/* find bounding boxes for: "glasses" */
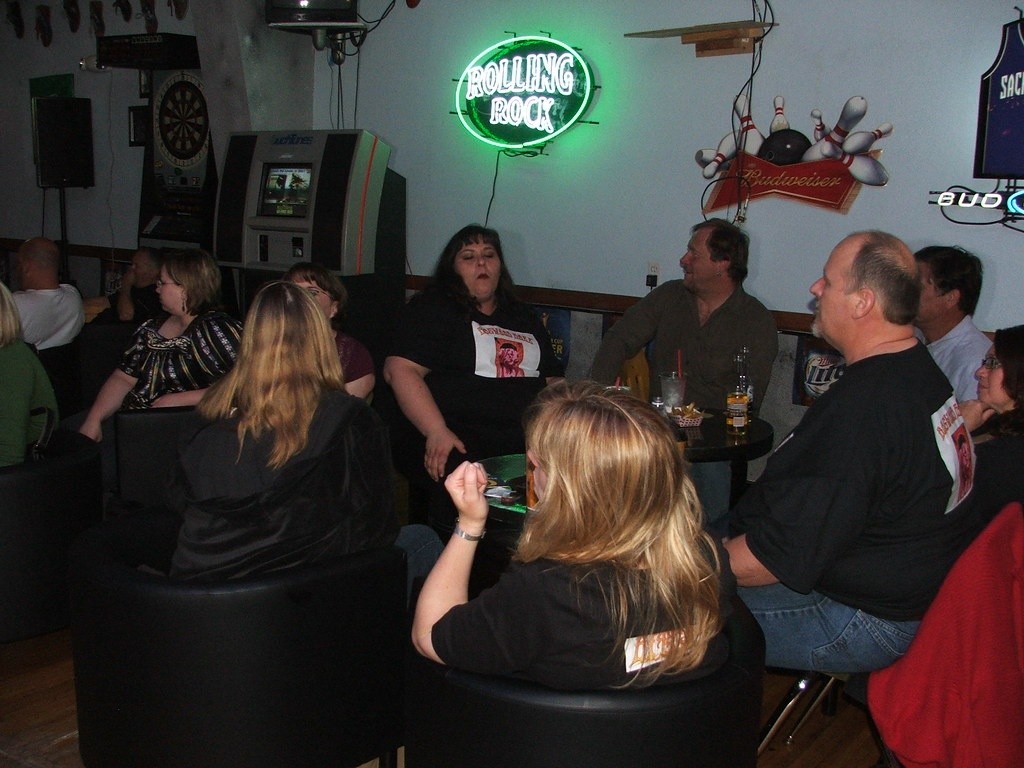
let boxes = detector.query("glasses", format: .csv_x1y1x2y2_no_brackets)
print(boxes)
306,288,333,299
980,357,1001,369
156,279,179,288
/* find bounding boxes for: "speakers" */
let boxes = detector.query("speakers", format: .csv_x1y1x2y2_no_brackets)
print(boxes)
32,96,96,188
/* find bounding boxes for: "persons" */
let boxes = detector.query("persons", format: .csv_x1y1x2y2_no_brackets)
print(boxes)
710,231,982,675
0,235,170,469
79,245,398,580
910,246,1024,505
411,381,736,693
383,223,572,485
585,218,779,522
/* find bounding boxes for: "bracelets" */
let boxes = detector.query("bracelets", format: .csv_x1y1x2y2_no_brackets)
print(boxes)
454,517,487,541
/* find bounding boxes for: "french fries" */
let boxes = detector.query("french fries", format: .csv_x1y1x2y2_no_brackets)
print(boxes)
673,402,705,419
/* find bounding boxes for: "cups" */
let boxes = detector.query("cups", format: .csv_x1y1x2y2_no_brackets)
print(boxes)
605,386,631,392
659,371,686,413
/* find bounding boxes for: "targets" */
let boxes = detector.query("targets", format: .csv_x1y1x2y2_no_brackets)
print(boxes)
158,80,209,158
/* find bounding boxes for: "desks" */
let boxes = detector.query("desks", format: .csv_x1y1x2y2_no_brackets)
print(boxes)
403,434,557,570
675,402,773,515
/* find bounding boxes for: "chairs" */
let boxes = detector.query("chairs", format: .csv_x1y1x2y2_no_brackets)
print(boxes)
1,323,1024,767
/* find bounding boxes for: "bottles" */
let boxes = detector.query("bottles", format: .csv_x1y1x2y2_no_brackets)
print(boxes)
726,355,748,436
652,397,669,421
740,346,753,422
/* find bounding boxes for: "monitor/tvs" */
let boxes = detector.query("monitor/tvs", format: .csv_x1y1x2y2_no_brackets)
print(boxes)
256,162,312,218
263,0,357,23
